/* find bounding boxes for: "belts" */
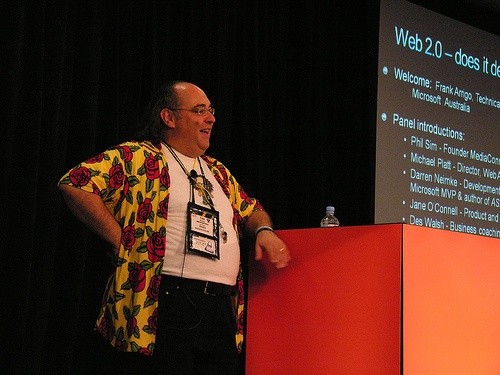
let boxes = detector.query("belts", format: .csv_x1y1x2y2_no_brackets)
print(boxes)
161,275,235,296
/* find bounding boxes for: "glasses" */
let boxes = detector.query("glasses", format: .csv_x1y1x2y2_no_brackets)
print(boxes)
170,106,215,116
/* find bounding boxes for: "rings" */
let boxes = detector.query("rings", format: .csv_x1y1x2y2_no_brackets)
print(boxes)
279,248,284,252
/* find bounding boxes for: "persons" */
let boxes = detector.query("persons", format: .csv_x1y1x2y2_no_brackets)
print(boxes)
57,78,291,375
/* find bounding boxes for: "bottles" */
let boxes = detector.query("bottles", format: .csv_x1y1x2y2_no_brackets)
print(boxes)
320,206,340,228
